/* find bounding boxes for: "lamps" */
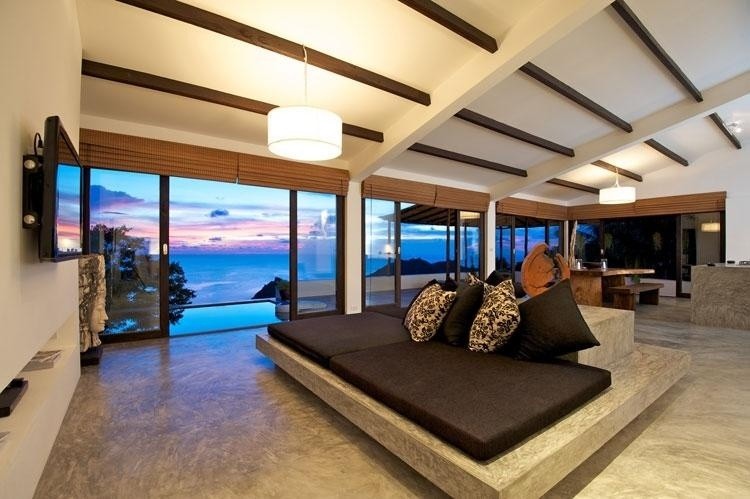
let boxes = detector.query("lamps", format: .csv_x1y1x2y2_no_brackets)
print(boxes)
701,213,720,233
266,45,344,165
599,168,637,205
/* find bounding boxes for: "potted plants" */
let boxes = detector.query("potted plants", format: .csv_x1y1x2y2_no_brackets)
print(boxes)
276,279,299,305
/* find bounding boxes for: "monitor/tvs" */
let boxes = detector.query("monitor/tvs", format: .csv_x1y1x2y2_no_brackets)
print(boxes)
20,114,88,265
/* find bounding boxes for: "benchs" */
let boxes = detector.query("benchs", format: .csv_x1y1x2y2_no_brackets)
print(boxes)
252,307,690,499
607,282,665,312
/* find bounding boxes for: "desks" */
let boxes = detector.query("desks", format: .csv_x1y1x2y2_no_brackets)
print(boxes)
569,268,656,306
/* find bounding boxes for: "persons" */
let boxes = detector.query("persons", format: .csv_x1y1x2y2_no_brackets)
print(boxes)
90,296,110,346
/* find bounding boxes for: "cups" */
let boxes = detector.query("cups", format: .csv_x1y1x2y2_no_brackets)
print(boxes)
576,259,583,270
601,259,608,272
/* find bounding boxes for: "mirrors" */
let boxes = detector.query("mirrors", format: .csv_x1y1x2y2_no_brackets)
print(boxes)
574,223,602,268
680,211,721,294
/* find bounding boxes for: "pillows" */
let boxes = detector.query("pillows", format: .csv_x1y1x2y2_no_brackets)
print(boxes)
405,271,602,362
